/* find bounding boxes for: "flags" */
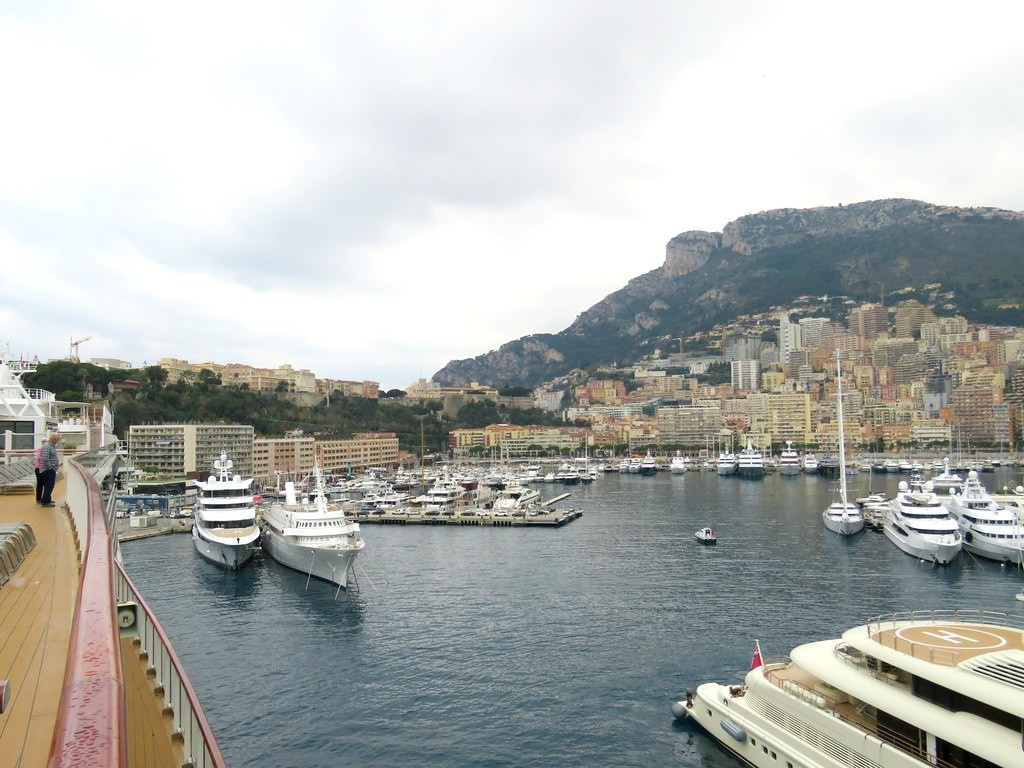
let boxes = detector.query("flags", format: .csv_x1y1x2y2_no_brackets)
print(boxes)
750,641,762,671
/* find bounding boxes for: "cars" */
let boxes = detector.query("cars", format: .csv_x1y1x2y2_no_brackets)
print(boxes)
372,509,386,515
392,508,404,515
494,510,508,516
563,510,570,515
539,506,556,515
116,506,194,518
569,506,575,511
512,510,526,517
406,509,419,516
424,509,440,515
530,510,539,516
441,510,455,515
476,511,490,516
460,511,475,516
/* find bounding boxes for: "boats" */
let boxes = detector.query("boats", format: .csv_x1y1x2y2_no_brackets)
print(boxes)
855,457,1024,565
677,607,1024,768
190,449,260,571
694,528,717,543
259,462,366,590
267,435,819,509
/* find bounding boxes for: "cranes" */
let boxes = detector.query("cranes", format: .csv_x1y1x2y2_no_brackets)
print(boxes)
71,335,92,364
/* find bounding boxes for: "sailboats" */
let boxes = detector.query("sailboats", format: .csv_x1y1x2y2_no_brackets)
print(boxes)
823,348,865,537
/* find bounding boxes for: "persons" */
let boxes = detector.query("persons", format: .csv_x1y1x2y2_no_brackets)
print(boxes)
35,433,61,508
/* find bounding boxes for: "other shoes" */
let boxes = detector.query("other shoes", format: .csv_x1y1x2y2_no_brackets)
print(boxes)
36,499,44,504
41,502,55,507
50,501,56,503
